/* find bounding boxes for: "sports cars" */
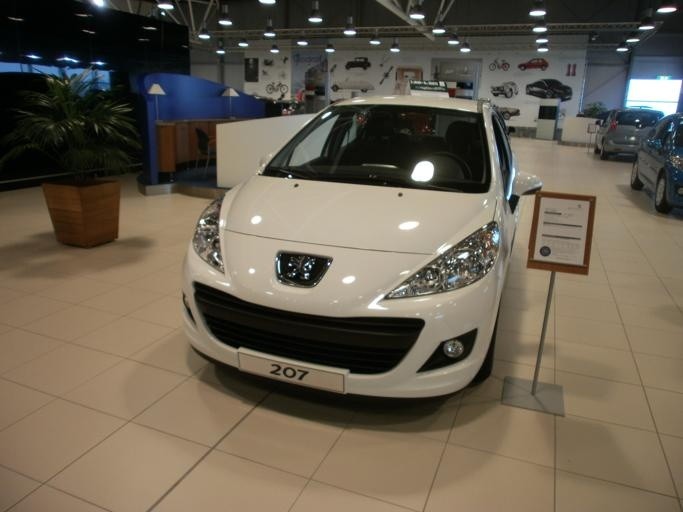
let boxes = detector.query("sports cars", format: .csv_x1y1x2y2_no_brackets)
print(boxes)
330,77,376,93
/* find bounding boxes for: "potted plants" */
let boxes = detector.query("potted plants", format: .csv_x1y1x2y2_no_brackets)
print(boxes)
0,63,144,249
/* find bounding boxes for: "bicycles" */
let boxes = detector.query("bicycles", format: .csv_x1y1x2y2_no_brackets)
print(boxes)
487,52,510,71
266,82,289,94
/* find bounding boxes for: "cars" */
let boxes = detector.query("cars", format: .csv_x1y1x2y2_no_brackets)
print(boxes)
519,58,549,71
492,105,520,119
304,59,325,96
629,112,683,216
526,78,572,102
180,92,542,398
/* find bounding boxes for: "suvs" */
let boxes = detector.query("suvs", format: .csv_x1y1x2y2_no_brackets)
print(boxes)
345,57,371,70
489,80,519,97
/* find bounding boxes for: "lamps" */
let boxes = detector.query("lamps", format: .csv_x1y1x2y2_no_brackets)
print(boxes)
220,88,241,119
147,83,168,122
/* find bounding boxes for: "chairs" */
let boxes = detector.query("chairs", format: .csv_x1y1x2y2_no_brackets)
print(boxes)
192,126,215,176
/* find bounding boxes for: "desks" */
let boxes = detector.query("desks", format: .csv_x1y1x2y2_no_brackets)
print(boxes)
155,117,256,183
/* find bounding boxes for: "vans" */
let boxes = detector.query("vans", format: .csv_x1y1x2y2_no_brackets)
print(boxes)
594,107,663,160
